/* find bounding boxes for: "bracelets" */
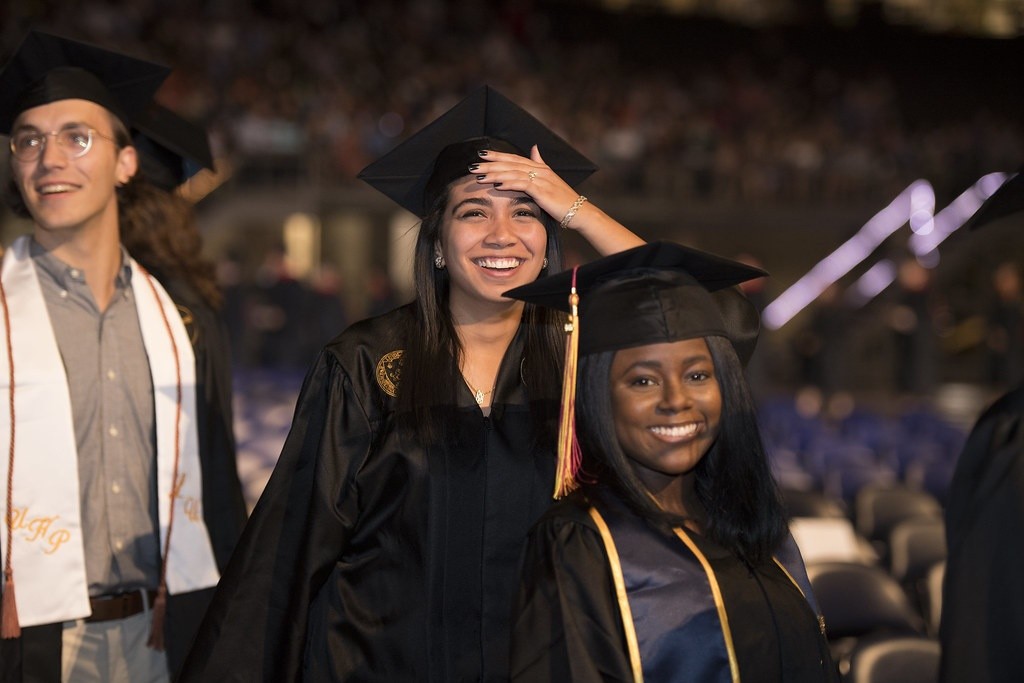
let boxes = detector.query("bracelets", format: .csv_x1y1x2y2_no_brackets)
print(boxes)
559,195,588,230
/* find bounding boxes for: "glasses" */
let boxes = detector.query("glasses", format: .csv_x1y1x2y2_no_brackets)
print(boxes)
9,125,120,162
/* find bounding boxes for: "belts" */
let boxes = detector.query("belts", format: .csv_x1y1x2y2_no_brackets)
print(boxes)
84,590,154,623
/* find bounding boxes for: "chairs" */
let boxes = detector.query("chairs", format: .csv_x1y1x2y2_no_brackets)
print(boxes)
230,367,311,520
753,399,975,683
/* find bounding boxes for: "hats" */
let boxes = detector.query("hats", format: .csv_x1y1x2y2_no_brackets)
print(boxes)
356,85,601,221
131,99,216,192
0,28,172,133
501,239,772,501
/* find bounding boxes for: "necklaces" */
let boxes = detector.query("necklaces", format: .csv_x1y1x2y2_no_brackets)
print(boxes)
461,373,496,402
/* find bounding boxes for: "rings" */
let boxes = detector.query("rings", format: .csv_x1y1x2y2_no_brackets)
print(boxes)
529,171,537,181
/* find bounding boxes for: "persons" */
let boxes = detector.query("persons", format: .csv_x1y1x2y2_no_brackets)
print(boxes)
501,240,837,683
0,30,248,682
185,80,646,683
